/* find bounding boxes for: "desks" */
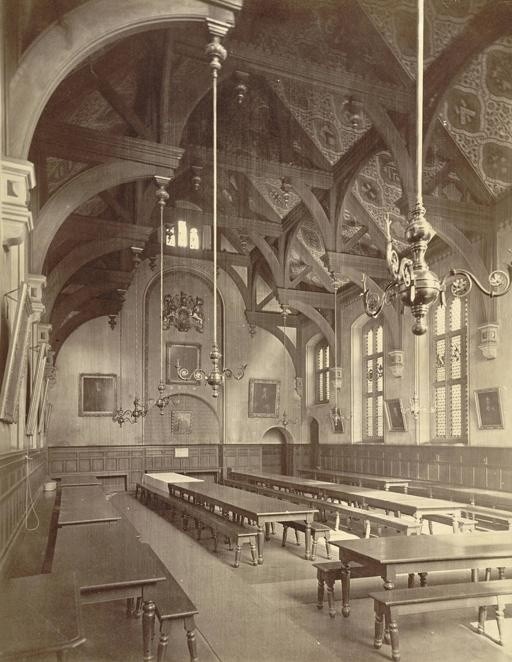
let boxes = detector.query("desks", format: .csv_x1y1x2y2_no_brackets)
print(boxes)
61,475,102,486
425,483,512,510
232,468,470,535
57,485,122,524
141,472,319,560
52,521,167,618
296,467,411,494
327,530,511,618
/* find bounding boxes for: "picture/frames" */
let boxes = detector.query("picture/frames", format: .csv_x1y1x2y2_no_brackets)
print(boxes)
329,408,344,433
384,398,408,432
166,341,201,387
25,343,48,436
78,373,118,417
0,281,34,424
473,387,505,430
248,378,280,419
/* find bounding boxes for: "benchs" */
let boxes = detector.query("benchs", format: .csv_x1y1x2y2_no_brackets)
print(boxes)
400,509,477,534
222,479,422,539
367,579,511,661
135,483,258,567
229,510,331,561
312,561,491,617
126,507,199,662
464,503,512,532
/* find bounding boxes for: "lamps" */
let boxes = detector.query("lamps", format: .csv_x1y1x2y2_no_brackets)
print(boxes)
36,320,53,344
294,376,304,400
387,346,405,378
475,321,500,363
329,366,343,392
25,274,48,304
